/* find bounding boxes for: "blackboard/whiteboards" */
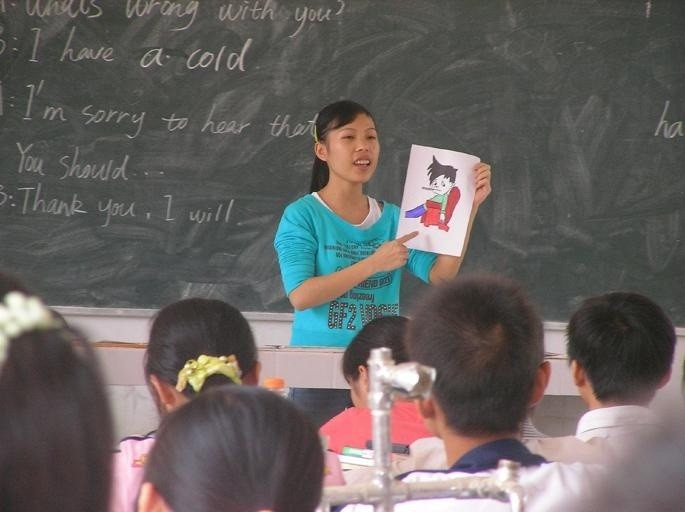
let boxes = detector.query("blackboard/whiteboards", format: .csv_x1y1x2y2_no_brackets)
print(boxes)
0,0,684,338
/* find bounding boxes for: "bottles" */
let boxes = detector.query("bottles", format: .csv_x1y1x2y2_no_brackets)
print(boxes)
262,378,289,398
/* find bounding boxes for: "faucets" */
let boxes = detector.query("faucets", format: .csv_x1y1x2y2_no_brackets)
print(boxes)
367,346,435,476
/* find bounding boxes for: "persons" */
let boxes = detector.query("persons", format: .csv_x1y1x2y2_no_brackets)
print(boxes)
111,296,263,512
524,291,677,462
0,273,117,511
273,98,493,427
318,314,434,455
405,155,458,224
135,383,325,512
333,273,610,511
